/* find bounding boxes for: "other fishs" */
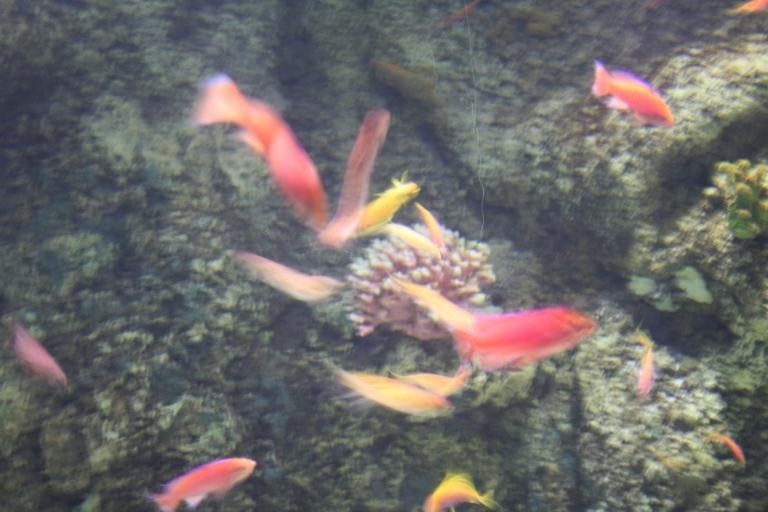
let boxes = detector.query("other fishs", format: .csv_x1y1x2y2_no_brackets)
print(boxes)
193,72,598,421
